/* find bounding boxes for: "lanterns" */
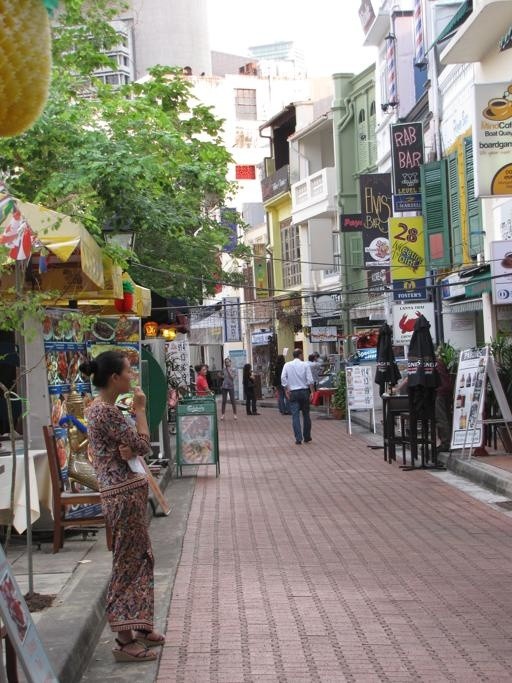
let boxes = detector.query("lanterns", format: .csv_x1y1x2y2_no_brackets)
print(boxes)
159,324,177,341
144,321,158,336
115,280,134,312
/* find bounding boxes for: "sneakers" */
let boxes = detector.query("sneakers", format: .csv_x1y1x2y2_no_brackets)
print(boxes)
295,437,312,444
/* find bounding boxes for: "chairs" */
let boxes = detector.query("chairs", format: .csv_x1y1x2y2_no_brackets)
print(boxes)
42,425,115,553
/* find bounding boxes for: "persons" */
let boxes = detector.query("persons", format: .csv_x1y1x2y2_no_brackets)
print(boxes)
195,365,215,396
220,358,238,420
272,355,292,415
306,352,325,393
243,364,260,415
397,358,454,452
281,348,314,444
79,351,166,662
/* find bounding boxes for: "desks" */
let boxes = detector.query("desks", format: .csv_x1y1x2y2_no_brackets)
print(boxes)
1,448,49,553
382,392,409,463
318,386,337,419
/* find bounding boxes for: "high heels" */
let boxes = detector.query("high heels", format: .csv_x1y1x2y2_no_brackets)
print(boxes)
133,630,167,648
112,637,158,663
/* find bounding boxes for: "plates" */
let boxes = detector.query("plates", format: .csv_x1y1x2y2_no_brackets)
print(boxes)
91,320,115,341
43,325,73,340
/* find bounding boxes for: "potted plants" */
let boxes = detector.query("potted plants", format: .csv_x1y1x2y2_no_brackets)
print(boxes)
330,370,346,420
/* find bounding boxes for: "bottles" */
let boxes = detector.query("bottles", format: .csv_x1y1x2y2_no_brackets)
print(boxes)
456,371,476,430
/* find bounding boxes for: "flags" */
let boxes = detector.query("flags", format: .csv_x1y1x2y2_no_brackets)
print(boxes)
0,180,50,275
